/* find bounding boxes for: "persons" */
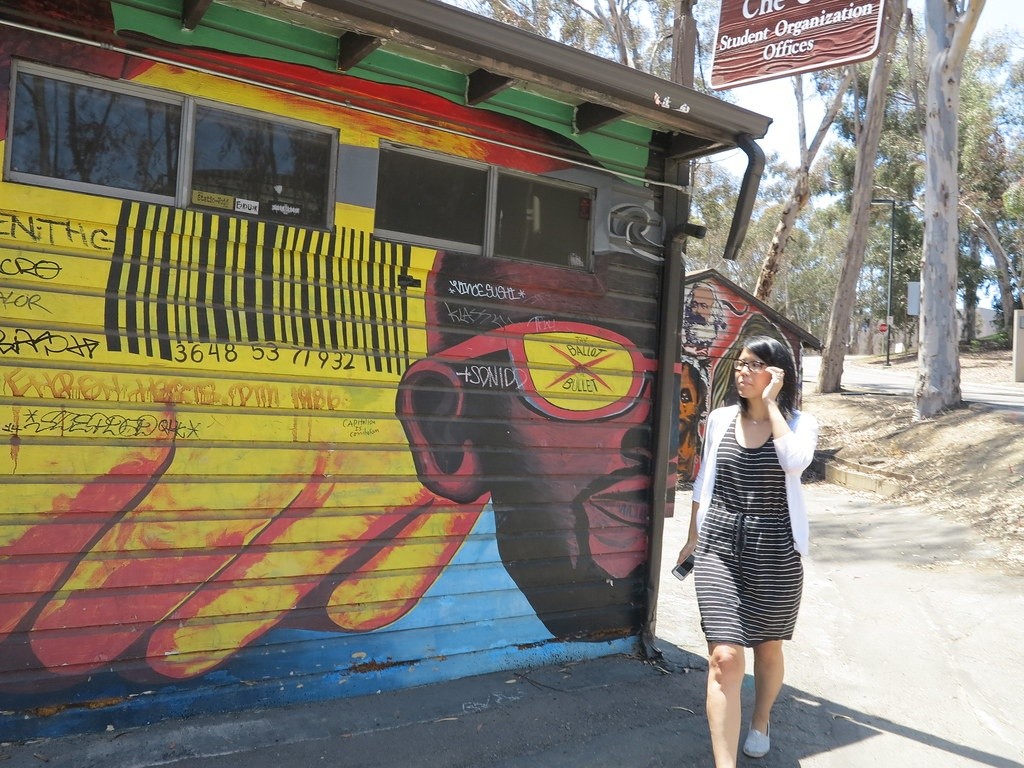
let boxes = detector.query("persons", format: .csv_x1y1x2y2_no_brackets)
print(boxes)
676,334,818,768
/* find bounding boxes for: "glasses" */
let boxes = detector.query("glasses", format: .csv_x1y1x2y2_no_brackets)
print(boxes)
733,358,768,372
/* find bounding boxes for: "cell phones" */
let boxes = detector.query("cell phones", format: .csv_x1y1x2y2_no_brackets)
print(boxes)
672,551,696,580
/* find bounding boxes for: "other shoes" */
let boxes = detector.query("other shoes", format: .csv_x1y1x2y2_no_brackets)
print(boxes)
743,716,771,758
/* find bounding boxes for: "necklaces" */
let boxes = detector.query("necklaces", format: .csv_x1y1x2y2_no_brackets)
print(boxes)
745,413,769,425
